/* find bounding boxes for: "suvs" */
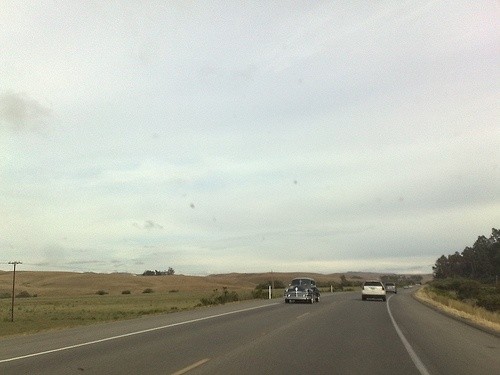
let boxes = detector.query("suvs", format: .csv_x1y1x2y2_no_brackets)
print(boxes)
362,280,386,300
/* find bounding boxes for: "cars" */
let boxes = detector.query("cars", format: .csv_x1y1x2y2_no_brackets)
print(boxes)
384,282,396,294
284,277,320,304
